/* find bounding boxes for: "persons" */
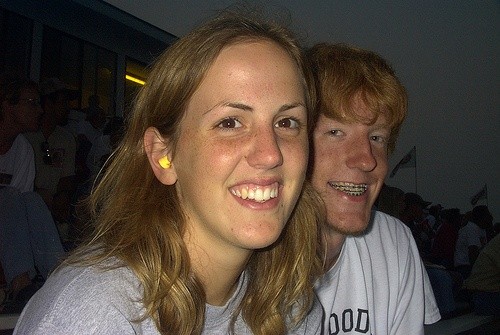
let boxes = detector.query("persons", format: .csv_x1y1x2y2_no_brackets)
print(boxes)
0,78,500,335
307,42,442,335
14,12,325,335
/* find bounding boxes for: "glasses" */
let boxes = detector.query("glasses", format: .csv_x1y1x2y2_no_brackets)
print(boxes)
12,98,42,106
41,141,52,165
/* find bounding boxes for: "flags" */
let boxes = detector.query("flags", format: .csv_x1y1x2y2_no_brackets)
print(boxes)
471,185,487,206
390,147,416,179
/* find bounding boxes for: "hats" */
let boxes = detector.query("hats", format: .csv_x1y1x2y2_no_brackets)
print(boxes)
405,193,432,207
